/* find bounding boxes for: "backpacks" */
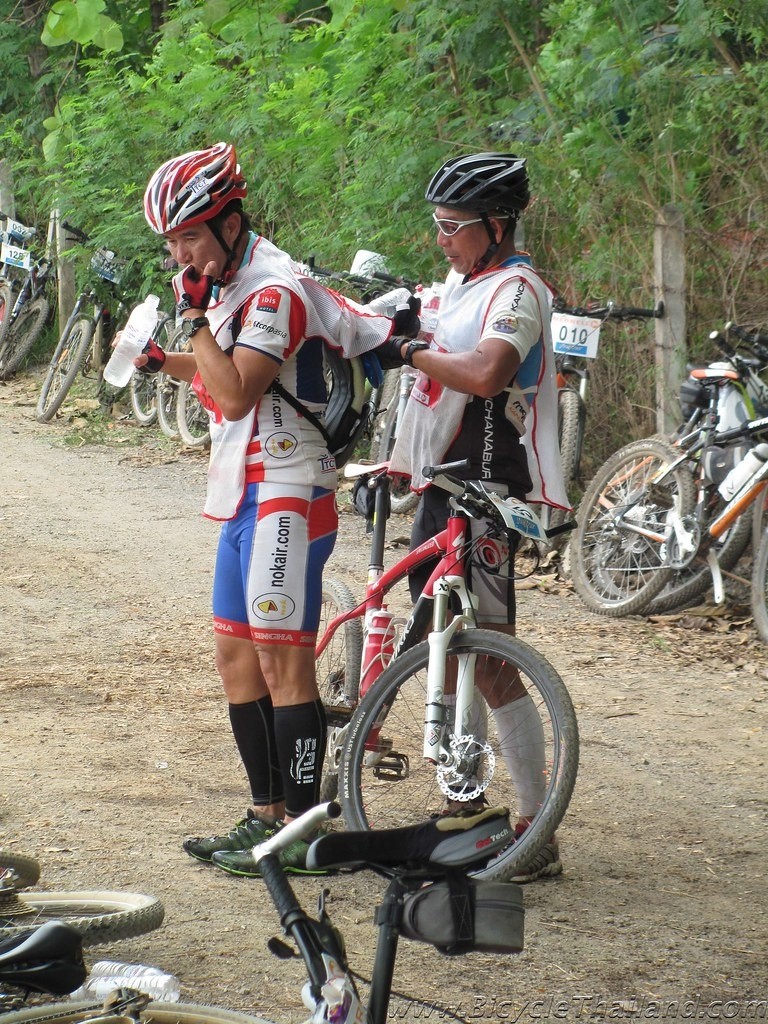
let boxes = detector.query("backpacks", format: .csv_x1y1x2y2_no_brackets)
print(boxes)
230,277,385,469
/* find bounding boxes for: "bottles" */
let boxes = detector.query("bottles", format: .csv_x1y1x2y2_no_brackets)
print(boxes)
718,442,768,501
70,961,181,1005
102,294,160,387
368,288,413,318
359,604,394,699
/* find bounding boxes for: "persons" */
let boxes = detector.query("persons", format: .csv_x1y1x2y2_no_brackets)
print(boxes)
112,141,340,877
377,151,572,882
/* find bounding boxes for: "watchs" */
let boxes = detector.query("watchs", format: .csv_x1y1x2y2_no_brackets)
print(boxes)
406,339,430,369
182,316,209,337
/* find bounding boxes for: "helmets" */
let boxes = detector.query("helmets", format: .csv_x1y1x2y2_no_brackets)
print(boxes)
142,140,249,260
425,152,531,285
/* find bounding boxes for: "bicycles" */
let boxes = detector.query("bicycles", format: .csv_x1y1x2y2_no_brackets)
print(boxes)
1,798,532,1023
307,455,579,884
0,216,767,640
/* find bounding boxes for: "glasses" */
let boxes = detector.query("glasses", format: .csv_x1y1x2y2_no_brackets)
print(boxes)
429,211,509,236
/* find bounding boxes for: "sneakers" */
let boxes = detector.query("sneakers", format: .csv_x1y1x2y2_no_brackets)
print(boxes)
430,809,451,820
498,824,563,882
183,808,340,878
